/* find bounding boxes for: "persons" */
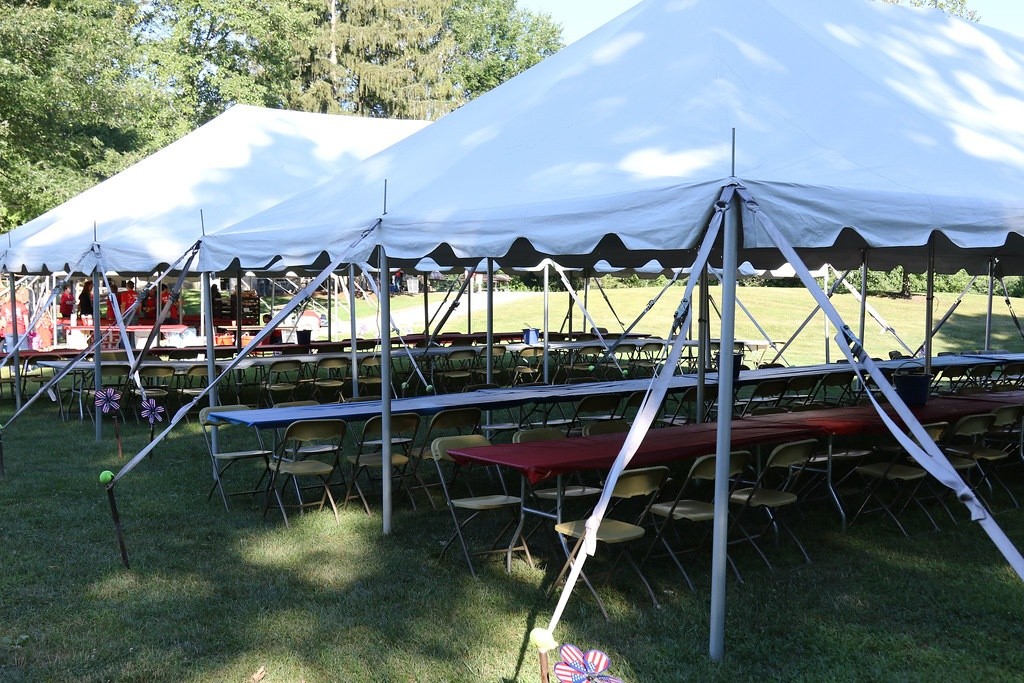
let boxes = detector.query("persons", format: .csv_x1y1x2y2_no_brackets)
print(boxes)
161,283,179,323
1,287,35,375
79,281,94,364
106,285,119,348
60,284,77,340
120,280,141,362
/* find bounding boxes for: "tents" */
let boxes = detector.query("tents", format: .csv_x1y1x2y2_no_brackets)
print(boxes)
197,0,1022,659
0,102,761,481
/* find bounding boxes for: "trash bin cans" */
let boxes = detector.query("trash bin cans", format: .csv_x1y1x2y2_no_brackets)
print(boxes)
6,333,29,372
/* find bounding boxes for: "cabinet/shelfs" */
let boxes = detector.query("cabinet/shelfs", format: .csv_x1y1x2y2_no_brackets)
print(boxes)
231,294,260,326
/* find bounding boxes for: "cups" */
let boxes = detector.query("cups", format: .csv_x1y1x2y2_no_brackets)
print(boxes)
232,320,236,326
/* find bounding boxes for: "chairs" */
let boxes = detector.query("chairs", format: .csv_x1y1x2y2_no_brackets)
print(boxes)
0,328,1024,624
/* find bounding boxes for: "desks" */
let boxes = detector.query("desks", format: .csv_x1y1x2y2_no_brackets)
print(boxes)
0,344,243,400
57,315,299,350
207,391,557,515
872,355,1007,390
446,420,815,570
35,358,254,422
391,341,549,371
947,390,1024,461
540,333,651,340
676,363,864,390
550,339,744,354
243,353,381,366
477,376,718,430
256,335,442,357
962,352,1024,385
442,332,540,341
745,398,1019,535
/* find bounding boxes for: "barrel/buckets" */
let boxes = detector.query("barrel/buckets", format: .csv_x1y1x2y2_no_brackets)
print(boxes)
522,328,541,346
216,333,257,347
406,278,420,294
296,330,312,344
